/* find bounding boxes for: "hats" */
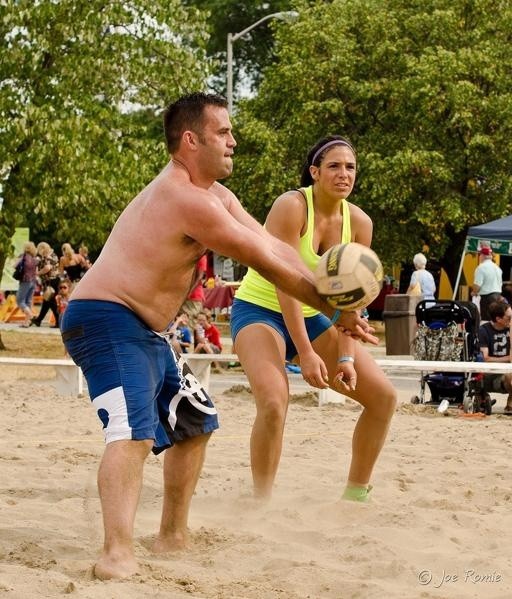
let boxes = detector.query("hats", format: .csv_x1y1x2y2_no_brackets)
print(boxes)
476,247,493,256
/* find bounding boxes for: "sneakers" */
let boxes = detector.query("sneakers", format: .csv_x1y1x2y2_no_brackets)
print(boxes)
19,315,60,329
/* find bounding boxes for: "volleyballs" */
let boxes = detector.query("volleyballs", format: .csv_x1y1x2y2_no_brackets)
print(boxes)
314,242,385,312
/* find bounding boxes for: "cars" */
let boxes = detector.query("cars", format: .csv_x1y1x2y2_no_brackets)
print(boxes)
367,271,400,320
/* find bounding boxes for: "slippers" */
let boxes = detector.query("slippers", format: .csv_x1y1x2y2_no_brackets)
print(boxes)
491,398,497,405
504,405,512,412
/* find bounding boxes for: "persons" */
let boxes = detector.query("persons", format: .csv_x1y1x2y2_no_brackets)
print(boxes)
472,247,512,411
167,254,223,370
15,242,93,331
227,132,399,507
58,92,382,581
406,253,436,309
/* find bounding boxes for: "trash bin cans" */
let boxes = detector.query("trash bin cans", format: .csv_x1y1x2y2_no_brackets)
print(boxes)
382,294,424,355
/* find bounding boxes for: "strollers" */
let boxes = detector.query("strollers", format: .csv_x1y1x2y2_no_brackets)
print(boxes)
407,297,498,417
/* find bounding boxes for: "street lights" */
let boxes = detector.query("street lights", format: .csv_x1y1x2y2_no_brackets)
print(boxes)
224,10,300,114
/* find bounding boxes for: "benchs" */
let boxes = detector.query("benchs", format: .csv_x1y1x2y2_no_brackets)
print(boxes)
174,353,510,422
1,354,87,399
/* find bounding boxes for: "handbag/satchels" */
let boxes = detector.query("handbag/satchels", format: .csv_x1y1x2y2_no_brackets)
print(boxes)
42,284,57,303
406,279,423,315
411,322,468,364
12,253,27,281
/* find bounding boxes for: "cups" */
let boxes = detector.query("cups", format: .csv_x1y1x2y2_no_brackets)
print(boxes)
480,348,489,358
